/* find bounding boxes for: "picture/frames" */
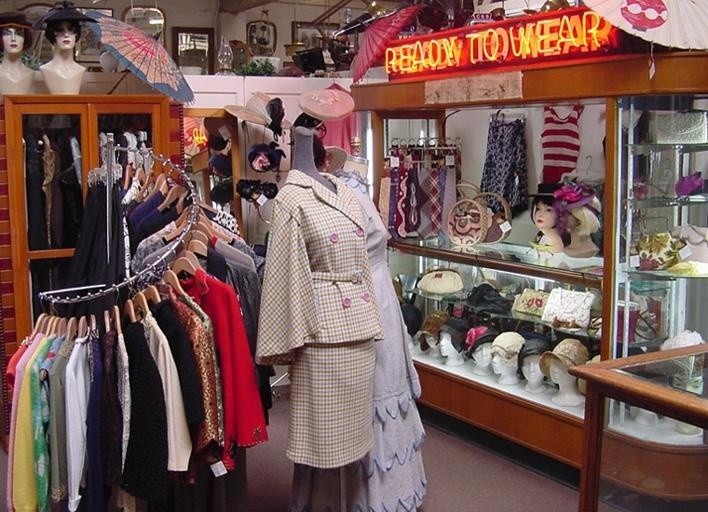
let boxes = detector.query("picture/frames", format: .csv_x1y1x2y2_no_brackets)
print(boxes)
292,21,340,48
69,8,114,65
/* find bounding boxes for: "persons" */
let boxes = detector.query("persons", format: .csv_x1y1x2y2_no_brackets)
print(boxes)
39,10,98,95
1,11,37,95
398,305,589,409
553,183,601,259
254,126,381,512
528,181,565,252
323,147,430,512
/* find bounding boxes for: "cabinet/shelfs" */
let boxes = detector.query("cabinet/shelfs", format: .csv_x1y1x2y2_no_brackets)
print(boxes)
2,91,171,344
568,342,706,509
350,49,707,500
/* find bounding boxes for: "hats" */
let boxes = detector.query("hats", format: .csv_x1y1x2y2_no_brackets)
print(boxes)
0,12,34,29
538,338,589,378
313,139,347,173
518,336,552,380
463,323,500,357
529,181,565,203
398,301,470,353
44,8,100,24
578,355,602,396
224,89,292,132
490,331,525,359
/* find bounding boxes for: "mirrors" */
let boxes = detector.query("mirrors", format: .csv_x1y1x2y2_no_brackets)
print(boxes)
171,26,215,76
181,107,246,240
120,6,166,49
246,21,277,58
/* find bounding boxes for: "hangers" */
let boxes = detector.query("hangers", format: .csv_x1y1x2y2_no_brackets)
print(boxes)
6,258,215,384
114,143,256,277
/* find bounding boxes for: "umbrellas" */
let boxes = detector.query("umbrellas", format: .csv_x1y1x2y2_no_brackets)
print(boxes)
351,5,424,82
84,10,196,104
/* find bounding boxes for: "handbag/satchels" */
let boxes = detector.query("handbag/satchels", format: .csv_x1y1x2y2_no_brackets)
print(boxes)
668,221,707,265
466,280,513,313
636,218,680,271
615,299,656,346
539,286,595,337
648,108,708,146
509,286,550,323
